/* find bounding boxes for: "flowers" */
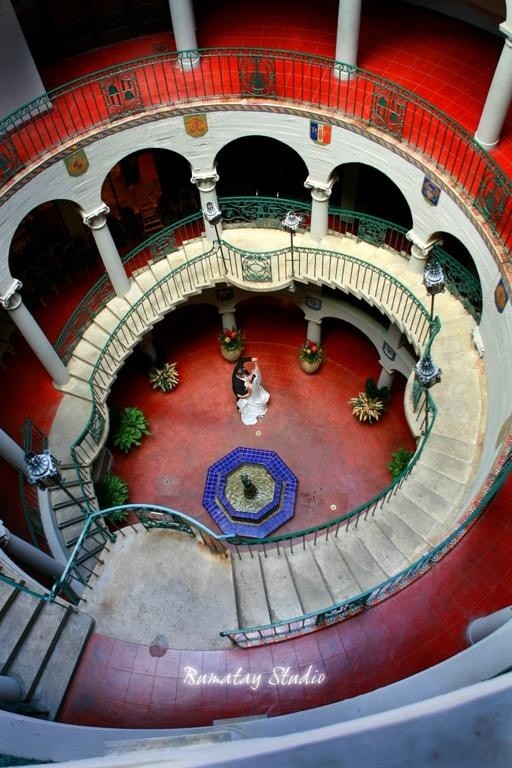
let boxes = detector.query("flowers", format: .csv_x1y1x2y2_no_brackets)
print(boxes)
217,328,244,352
298,338,322,363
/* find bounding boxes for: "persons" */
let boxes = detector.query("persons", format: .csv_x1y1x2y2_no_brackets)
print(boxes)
232,355,258,407
234,362,271,426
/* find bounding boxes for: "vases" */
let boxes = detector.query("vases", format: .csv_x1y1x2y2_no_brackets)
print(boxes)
300,358,320,374
220,344,241,362
